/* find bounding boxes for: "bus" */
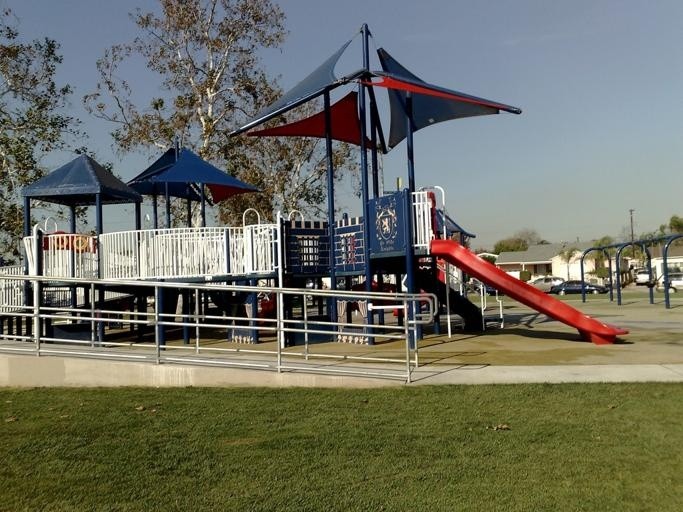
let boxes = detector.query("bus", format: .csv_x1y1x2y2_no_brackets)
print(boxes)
655,257,682,293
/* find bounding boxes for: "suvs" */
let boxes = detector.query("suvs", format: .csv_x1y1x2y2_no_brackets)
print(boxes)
527,276,564,294
635,270,653,285
655,272,682,294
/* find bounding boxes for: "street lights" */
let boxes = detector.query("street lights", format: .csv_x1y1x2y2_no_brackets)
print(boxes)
629,208,634,260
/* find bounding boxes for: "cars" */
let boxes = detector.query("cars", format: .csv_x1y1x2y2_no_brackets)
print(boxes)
484,284,500,294
550,280,608,295
